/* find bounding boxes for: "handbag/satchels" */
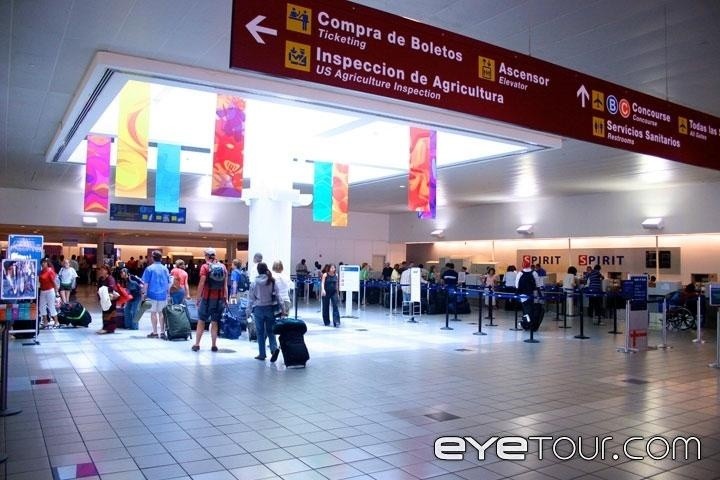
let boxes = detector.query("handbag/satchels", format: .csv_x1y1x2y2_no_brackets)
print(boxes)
108,290,120,301
170,275,180,293
271,317,307,335
57,313,69,325
271,279,290,323
217,302,241,340
115,282,134,305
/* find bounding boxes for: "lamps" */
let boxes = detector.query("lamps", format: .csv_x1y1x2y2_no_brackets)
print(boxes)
430,229,445,239
82,216,98,226
641,218,664,230
199,222,214,231
516,225,533,235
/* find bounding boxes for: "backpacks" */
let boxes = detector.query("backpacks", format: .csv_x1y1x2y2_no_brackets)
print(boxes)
518,270,536,295
237,270,251,292
205,261,226,290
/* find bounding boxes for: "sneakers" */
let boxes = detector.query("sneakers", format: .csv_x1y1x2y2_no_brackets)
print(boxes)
192,345,200,352
255,355,265,360
160,332,166,338
270,348,279,362
146,332,159,338
212,346,218,351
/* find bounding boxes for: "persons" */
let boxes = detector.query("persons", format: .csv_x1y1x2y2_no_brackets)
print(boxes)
39,246,655,362
666,283,697,320
3,261,20,297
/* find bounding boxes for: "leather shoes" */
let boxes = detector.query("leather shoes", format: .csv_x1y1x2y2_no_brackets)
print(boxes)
96,329,114,333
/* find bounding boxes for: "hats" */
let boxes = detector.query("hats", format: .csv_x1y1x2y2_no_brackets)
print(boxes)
203,247,217,258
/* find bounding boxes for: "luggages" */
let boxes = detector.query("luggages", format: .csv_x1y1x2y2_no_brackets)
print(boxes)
225,302,248,331
162,298,193,340
115,307,125,328
279,313,310,368
247,319,267,342
55,291,92,328
520,303,545,331
